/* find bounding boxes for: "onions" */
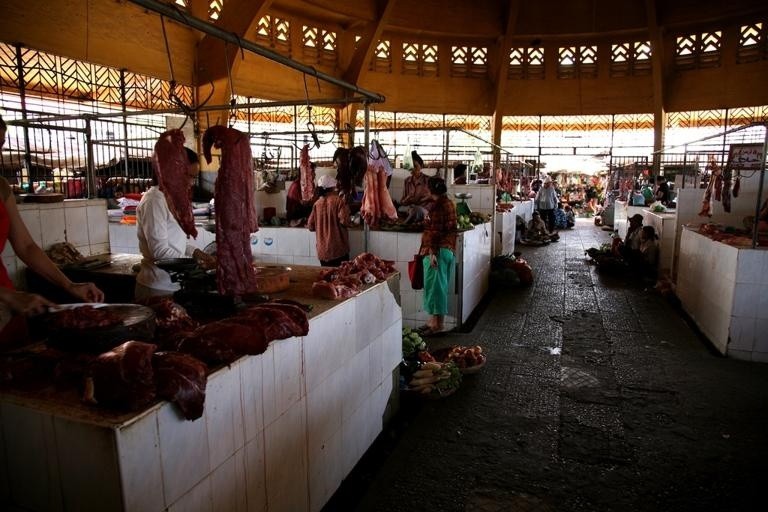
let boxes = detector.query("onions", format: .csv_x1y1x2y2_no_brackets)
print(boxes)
445,344,483,365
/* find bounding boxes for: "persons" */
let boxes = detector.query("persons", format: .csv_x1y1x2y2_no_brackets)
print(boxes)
622,214,643,251
654,176,670,203
534,176,560,234
408,175,457,337
134,147,216,306
626,225,660,297
286,163,325,219
527,211,560,242
0,116,105,332
553,202,568,230
307,175,356,267
400,150,437,212
564,205,575,228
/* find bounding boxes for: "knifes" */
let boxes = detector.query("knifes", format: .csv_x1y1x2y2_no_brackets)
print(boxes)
43,305,73,314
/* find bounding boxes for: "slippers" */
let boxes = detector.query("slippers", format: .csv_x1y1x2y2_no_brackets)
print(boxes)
418,323,448,338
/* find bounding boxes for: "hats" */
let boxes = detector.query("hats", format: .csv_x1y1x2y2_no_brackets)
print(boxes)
317,175,338,189
627,213,644,227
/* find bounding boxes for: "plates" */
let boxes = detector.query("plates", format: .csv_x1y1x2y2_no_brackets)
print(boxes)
18,192,66,203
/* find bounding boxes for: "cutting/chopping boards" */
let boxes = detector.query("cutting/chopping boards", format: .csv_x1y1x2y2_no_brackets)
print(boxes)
57,303,157,342
254,267,290,294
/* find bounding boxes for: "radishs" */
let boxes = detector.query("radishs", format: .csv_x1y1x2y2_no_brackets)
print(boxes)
409,361,452,394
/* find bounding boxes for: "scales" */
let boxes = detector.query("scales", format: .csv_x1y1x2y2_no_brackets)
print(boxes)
151,257,234,319
455,193,472,216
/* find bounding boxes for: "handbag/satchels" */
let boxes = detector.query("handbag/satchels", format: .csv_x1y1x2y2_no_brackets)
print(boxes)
407,242,424,290
